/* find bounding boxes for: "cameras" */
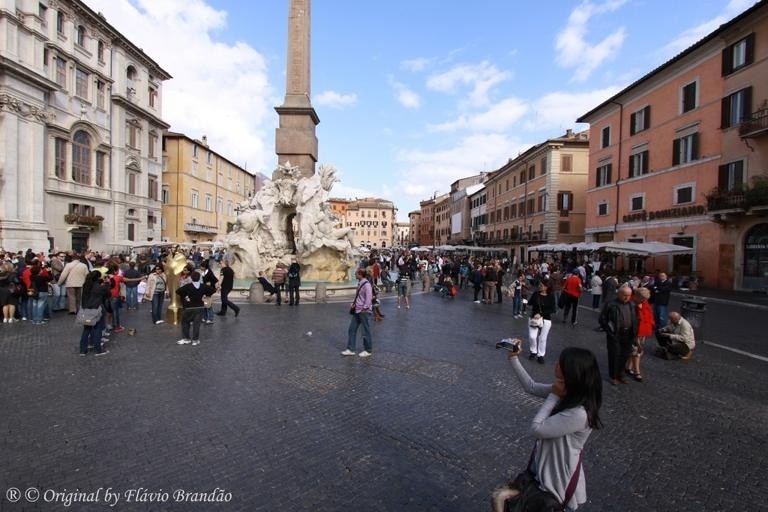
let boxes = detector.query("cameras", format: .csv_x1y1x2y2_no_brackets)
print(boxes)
496,338,519,353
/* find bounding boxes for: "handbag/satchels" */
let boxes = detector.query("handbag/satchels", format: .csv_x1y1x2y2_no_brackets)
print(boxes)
74,306,103,326
491,469,565,512
349,304,356,315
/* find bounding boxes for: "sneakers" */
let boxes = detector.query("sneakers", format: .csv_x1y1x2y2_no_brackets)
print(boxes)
610,375,632,385
472,298,502,305
528,353,545,364
93,350,110,357
2,316,49,327
405,305,409,309
79,349,90,357
340,348,356,357
396,305,401,309
87,324,125,349
624,367,644,382
176,338,192,345
216,311,226,316
192,339,200,346
201,317,215,324
512,310,528,321
234,307,240,317
155,319,165,325
51,308,78,315
358,350,372,357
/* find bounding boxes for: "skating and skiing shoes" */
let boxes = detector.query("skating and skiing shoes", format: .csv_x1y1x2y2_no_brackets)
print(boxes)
373,313,385,321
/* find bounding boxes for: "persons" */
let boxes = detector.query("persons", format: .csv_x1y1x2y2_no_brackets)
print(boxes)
0,245,240,356
289,258,301,305
283,264,291,303
598,285,640,385
341,268,373,357
492,337,602,511
657,311,696,360
271,263,285,306
257,271,276,303
527,279,556,362
228,165,356,280
625,286,656,382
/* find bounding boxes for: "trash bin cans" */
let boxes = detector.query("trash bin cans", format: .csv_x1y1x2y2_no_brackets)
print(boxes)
680,299,708,344
